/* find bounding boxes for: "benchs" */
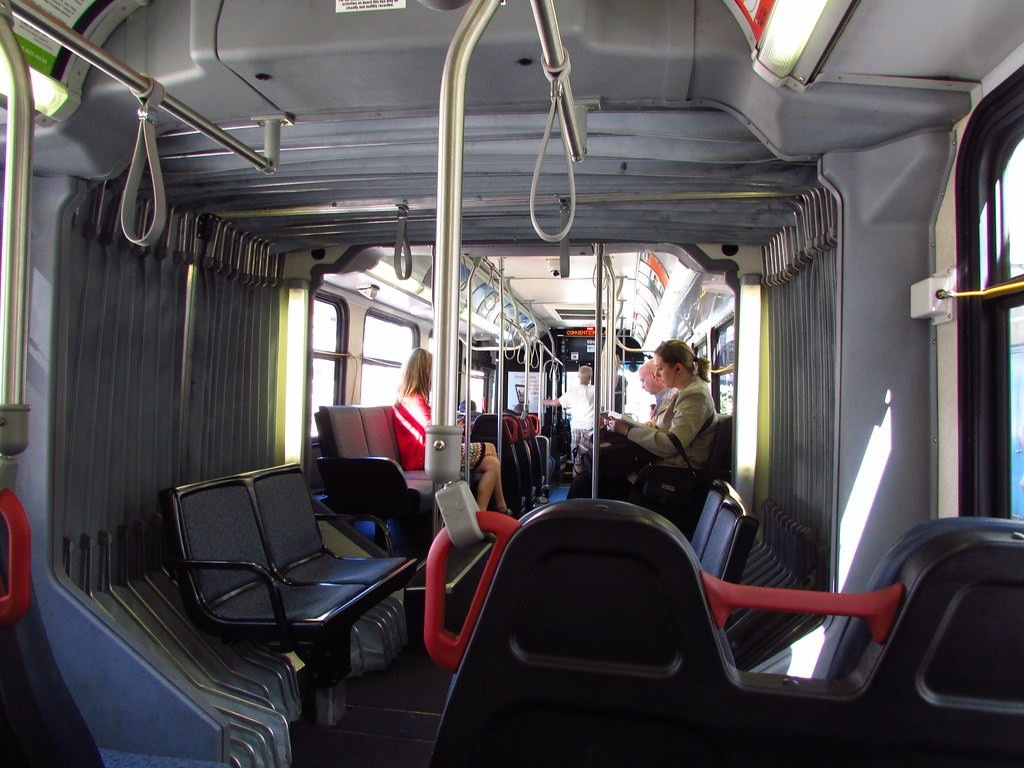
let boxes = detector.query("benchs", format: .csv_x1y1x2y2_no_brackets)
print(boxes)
162,412,1024,768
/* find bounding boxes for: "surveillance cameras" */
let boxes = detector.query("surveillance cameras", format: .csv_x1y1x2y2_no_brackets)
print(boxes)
551,270,559,277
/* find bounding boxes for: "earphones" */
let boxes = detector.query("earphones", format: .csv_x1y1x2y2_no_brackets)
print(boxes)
676,367,680,371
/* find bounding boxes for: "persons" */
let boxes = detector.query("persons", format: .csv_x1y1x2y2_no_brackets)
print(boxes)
543,366,595,477
566,340,718,498
459,400,476,412
514,403,524,413
615,351,627,414
391,348,513,517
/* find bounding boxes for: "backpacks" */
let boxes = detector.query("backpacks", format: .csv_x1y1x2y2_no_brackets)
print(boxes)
572,425,654,480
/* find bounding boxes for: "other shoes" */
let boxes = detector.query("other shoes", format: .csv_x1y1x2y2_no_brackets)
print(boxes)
538,496,549,504
484,532,496,544
494,506,513,517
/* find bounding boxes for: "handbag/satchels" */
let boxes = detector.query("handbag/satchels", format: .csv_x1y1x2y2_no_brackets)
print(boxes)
644,433,701,497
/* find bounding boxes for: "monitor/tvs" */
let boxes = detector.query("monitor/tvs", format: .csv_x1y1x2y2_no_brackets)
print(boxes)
515,384,530,403
